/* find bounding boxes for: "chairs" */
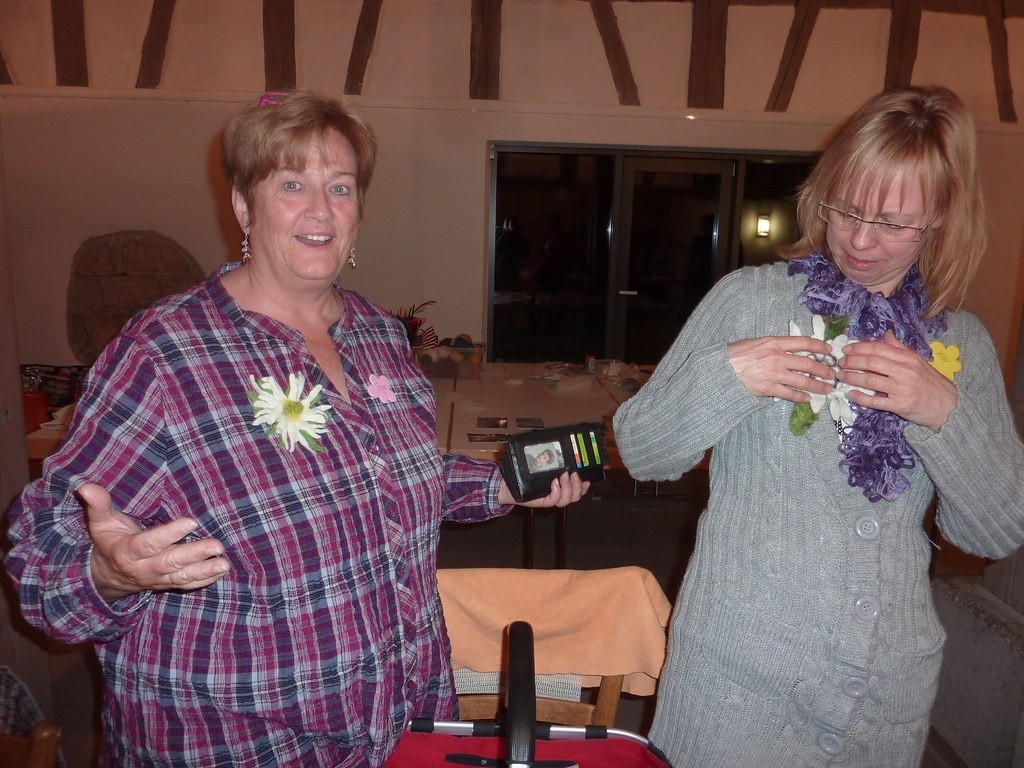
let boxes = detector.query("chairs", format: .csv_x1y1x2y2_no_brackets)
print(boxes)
433,563,648,730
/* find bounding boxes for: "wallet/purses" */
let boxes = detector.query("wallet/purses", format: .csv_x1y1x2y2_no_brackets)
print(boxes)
497,423,607,503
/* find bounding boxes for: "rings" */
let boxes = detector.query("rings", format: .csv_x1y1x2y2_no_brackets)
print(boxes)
557,503,561,508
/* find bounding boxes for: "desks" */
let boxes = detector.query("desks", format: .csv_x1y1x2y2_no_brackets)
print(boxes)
429,363,713,570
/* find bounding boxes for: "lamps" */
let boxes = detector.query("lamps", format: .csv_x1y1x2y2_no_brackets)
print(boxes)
757,213,772,237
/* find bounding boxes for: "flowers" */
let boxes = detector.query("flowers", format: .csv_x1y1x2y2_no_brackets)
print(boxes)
243,372,334,454
783,316,876,433
927,342,963,385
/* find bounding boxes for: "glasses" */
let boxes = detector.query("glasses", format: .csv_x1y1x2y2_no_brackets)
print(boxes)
818,190,942,243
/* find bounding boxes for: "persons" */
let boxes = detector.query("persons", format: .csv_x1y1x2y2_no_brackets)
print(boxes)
0,91,590,768
613,87,1024,768
525,450,556,473
